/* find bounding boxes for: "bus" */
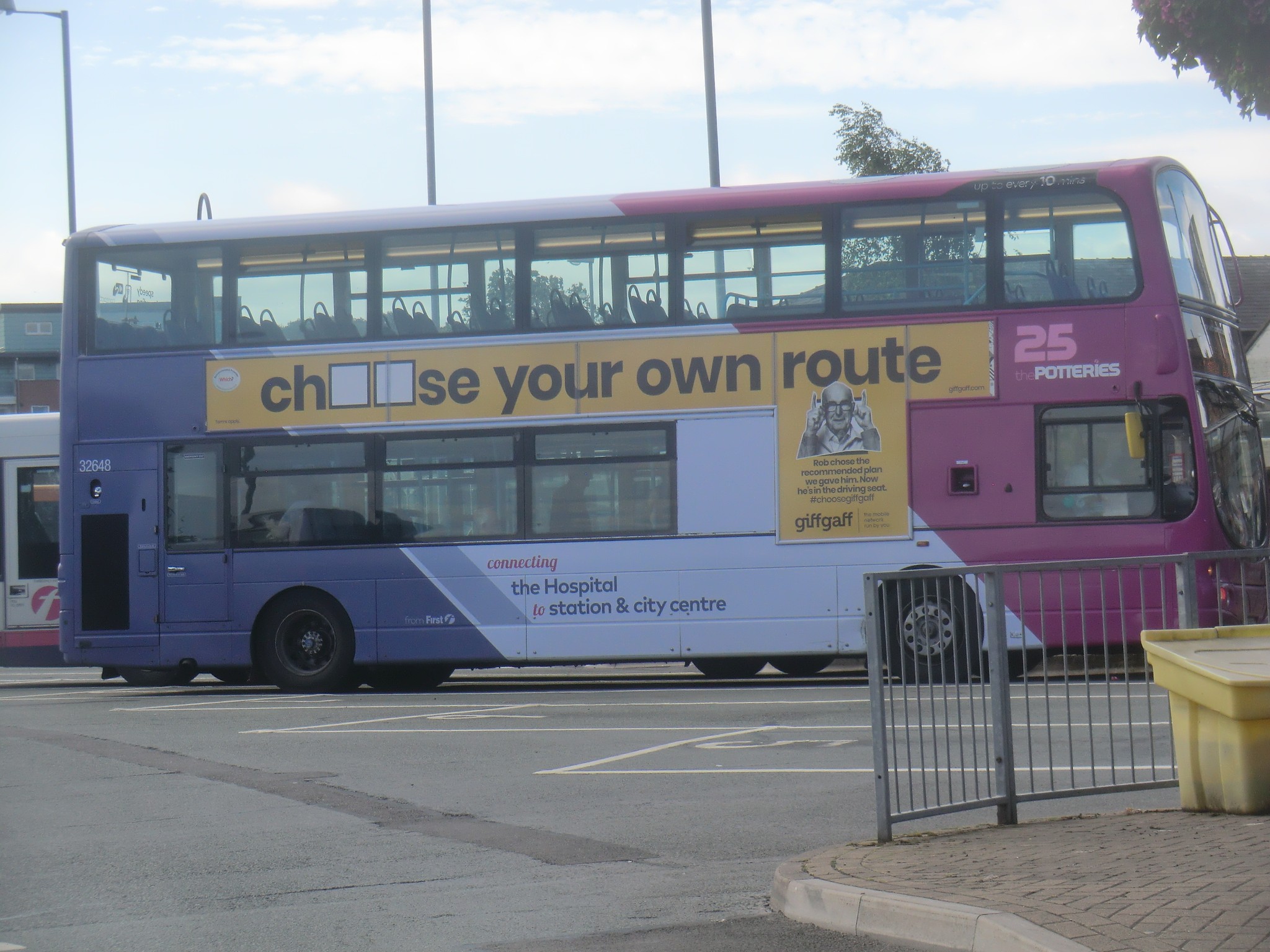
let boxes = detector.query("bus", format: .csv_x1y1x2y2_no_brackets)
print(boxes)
46,152,1270,702
0,411,64,666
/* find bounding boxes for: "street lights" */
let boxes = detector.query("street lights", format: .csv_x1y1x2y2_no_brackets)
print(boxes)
1,0,76,238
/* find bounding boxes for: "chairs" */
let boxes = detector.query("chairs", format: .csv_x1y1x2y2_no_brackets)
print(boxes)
93,279,1107,352
241,497,665,542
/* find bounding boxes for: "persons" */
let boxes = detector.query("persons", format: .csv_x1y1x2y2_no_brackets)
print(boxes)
797,381,881,459
1063,437,1136,519
